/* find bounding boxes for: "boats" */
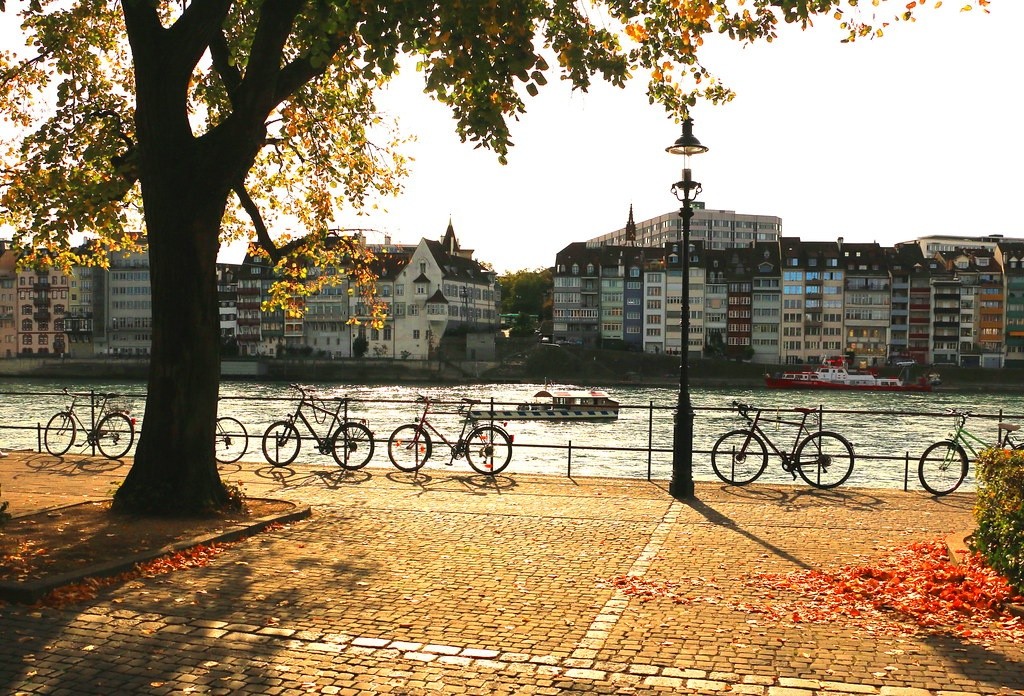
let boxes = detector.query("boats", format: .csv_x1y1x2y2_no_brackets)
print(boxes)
764,355,942,393
461,376,620,421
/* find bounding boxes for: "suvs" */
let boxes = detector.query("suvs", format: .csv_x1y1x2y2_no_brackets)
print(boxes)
555,336,583,347
895,359,916,367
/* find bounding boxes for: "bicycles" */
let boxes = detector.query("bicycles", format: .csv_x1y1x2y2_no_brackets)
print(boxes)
711,400,855,490
214,397,248,465
44,387,137,461
388,393,515,476
261,383,375,471
917,408,1024,496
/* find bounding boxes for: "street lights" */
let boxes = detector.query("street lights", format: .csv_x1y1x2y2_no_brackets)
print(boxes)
663,112,709,498
